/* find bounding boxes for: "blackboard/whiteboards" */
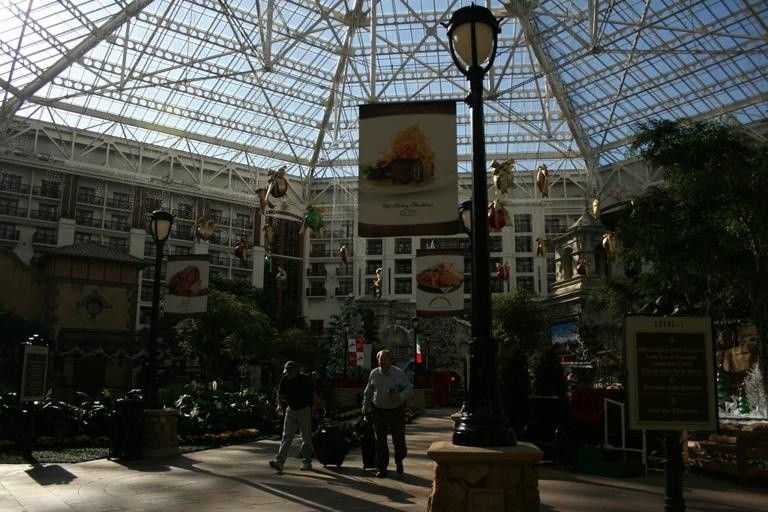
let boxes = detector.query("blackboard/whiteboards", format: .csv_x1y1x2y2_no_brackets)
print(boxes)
603,397,626,450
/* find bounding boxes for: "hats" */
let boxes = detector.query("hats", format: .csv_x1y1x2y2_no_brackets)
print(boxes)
283,361,297,373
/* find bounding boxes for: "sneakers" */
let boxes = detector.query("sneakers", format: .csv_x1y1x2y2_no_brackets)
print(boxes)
269,459,283,472
299,463,312,470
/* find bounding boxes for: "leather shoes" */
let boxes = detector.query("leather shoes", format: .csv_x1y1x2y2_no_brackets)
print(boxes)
397,465,403,474
376,471,388,478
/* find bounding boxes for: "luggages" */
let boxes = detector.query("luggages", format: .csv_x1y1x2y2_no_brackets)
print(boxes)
311,414,347,467
362,441,378,471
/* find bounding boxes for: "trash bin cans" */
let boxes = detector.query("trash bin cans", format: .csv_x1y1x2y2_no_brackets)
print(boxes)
110,399,145,462
453,412,492,443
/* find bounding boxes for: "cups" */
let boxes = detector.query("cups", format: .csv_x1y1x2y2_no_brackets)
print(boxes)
386,387,396,401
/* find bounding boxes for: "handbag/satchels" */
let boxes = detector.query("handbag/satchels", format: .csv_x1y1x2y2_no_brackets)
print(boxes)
352,413,375,443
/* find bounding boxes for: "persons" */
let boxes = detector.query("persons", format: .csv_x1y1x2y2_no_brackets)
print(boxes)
362,349,413,477
270,361,313,471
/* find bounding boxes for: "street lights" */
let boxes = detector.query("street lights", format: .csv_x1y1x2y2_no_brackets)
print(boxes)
337,319,351,387
138,204,178,409
458,185,478,247
433,0,528,446
